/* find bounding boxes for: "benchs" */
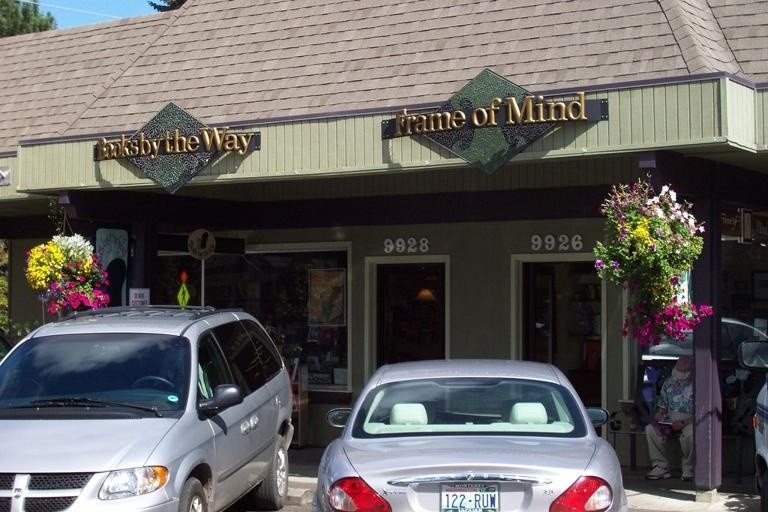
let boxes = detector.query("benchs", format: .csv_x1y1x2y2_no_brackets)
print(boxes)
609,405,756,485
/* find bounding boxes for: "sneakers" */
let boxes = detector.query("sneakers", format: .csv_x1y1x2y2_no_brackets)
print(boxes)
646,465,671,480
680,472,694,480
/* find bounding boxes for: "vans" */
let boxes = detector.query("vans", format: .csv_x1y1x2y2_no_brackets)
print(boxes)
0,300,295,511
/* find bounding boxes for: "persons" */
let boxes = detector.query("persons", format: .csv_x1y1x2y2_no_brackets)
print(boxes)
645,354,696,480
148,338,192,393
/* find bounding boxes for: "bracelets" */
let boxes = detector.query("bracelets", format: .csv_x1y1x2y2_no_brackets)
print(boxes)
680,420,689,426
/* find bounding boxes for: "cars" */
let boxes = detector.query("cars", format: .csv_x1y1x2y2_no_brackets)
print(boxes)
316,358,629,512
647,309,767,394
738,336,768,512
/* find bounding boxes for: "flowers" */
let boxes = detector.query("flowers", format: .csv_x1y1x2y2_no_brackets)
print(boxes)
593,169,716,350
21,230,113,319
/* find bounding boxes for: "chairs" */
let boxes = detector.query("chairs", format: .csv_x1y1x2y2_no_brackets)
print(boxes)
509,400,549,426
389,402,428,425
